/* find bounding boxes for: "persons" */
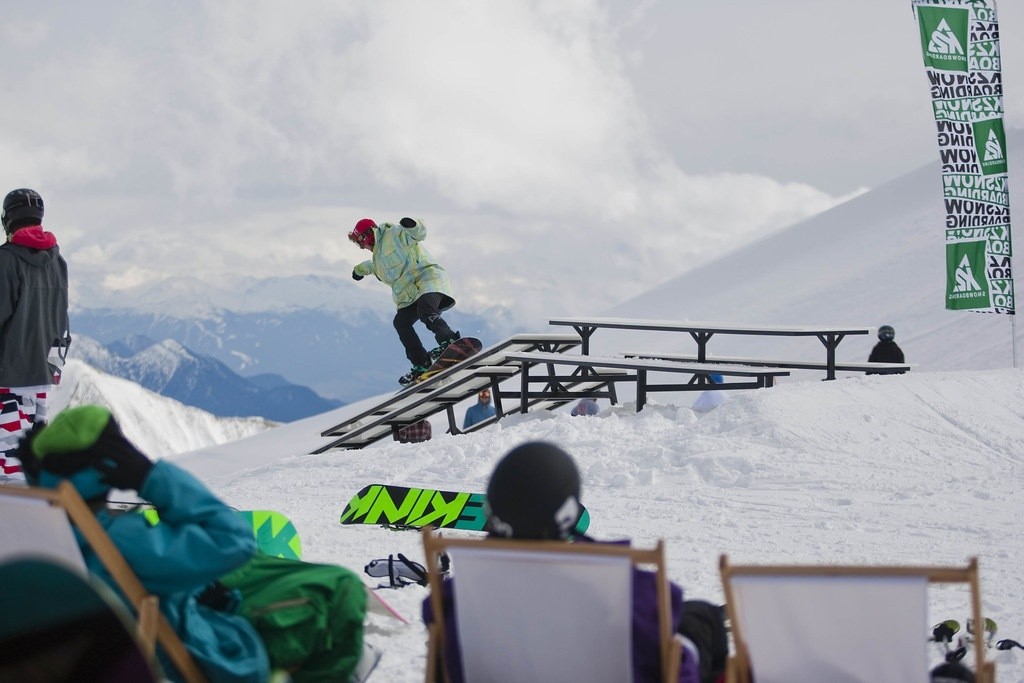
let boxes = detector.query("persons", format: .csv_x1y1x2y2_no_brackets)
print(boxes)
865,326,909,374
347,218,466,385
570,387,599,416
463,388,497,429
421,440,729,683
29,407,366,683
0,187,73,485
705,373,723,386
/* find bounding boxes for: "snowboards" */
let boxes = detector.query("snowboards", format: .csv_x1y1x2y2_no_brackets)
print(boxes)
135,508,304,561
391,336,482,396
339,482,590,542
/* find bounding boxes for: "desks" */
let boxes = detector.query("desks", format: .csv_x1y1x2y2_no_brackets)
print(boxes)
547,318,869,382
320,334,581,436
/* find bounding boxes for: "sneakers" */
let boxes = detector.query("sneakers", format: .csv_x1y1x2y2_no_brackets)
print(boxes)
398,361,431,383
430,332,460,359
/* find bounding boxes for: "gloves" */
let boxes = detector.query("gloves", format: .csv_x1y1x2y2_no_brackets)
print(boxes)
352,271,364,281
106,436,154,493
400,218,416,228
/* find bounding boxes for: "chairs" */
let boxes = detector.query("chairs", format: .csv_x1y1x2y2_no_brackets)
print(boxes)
0,482,208,683
718,553,996,682
420,525,684,682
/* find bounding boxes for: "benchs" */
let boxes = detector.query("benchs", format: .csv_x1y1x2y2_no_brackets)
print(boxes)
625,353,911,376
461,369,627,433
309,365,514,456
506,352,790,414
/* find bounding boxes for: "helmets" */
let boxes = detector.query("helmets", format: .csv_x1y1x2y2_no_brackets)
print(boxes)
877,326,895,342
491,440,581,542
351,218,377,247
1,189,44,233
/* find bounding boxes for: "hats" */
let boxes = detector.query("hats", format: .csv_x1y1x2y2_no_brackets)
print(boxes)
34,407,115,500
